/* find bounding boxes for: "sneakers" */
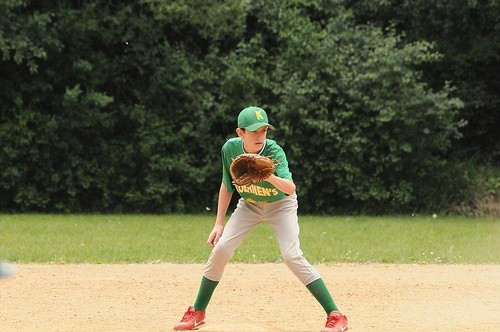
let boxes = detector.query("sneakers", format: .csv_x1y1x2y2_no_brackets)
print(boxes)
320,312,349,332
174,305,207,331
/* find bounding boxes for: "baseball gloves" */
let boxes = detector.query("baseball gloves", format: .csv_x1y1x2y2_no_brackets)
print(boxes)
230,153,274,185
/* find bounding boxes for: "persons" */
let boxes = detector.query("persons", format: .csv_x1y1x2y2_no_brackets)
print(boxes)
172,105,350,331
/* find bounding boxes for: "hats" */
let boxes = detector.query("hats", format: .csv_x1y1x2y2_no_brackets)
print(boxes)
238,106,275,131
